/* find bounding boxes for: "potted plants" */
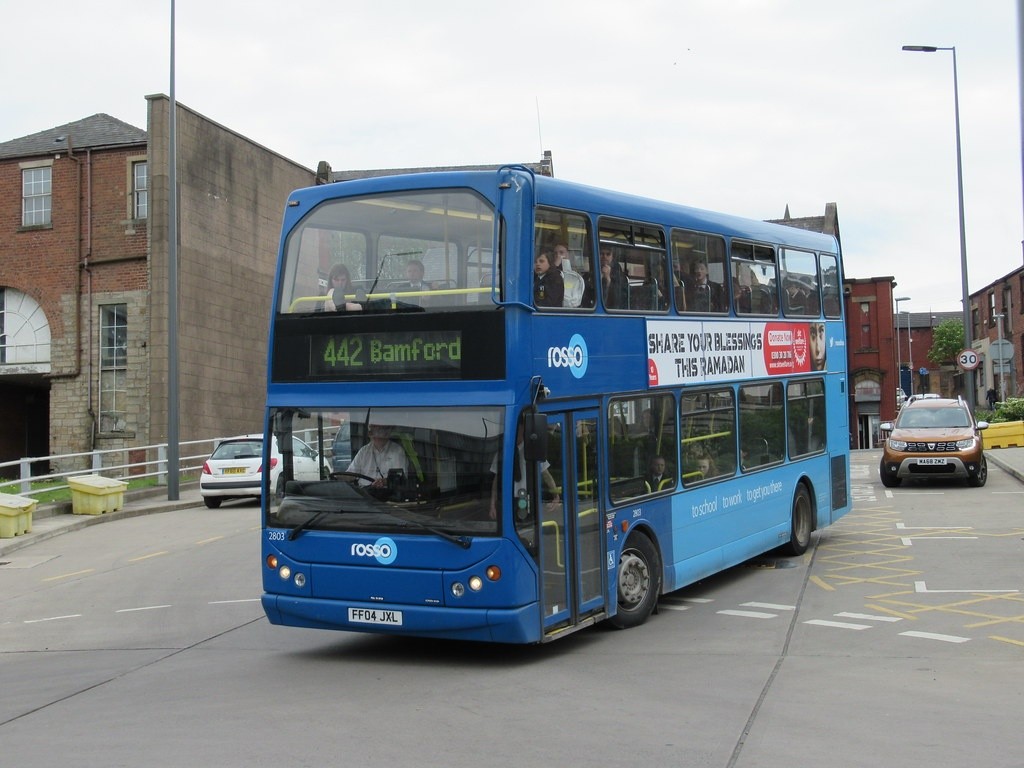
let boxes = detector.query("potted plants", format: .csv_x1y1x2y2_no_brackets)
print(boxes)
974,398,1024,450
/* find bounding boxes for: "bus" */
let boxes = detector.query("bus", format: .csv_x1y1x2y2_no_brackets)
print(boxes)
259,164,854,647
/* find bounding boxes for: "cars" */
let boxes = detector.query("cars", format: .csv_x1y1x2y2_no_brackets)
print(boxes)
199,431,333,509
901,393,942,407
896,387,907,410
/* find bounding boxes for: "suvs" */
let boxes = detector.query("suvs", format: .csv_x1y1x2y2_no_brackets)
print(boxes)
879,394,990,487
330,417,353,475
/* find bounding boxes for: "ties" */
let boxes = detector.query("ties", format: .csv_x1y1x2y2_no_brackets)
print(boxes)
513,445,521,483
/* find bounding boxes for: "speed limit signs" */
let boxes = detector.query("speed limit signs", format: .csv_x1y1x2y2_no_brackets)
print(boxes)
958,349,981,372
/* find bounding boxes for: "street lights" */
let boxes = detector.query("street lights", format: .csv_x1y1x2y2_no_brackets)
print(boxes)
992,314,1007,404
894,296,911,407
898,310,914,396
903,45,976,415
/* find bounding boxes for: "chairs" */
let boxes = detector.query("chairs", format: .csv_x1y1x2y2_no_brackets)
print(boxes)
740,437,769,469
240,445,254,455
350,272,841,317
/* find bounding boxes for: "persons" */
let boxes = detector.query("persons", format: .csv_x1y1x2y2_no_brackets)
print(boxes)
333,420,408,501
533,249,565,308
554,241,591,308
985,387,998,410
733,271,819,315
684,443,718,482
809,323,827,370
582,247,627,309
613,408,674,492
406,261,431,308
489,427,559,526
643,258,726,313
326,266,365,310
